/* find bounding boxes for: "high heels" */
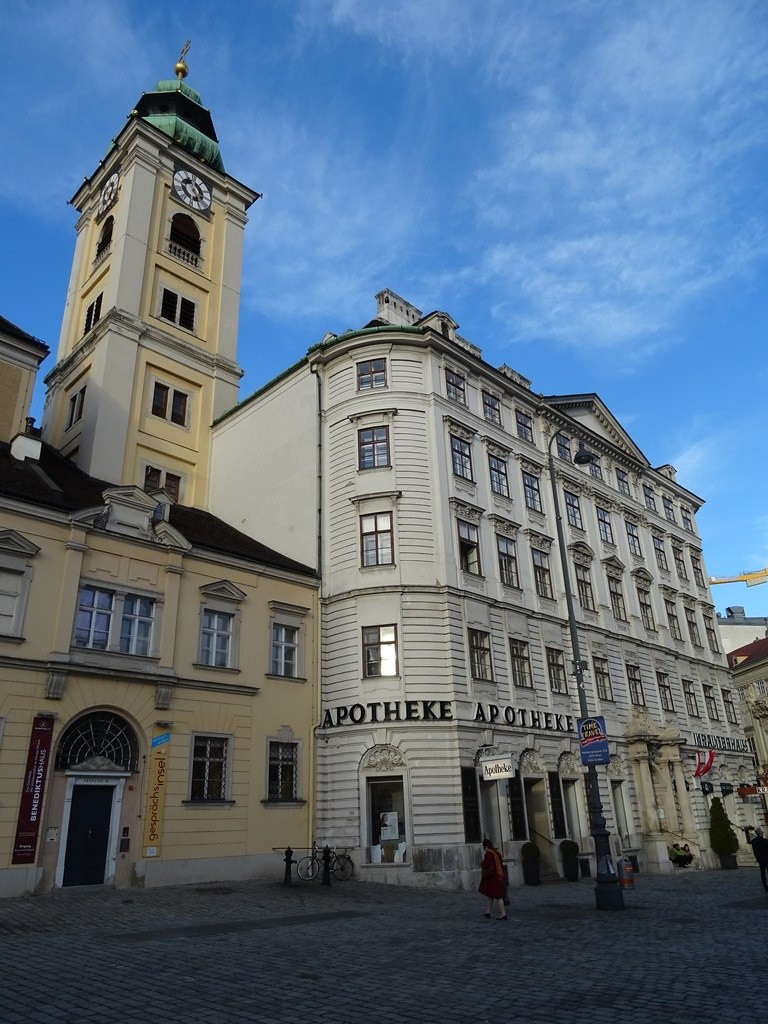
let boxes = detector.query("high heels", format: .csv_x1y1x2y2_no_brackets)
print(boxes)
484,913,490,917
497,915,507,920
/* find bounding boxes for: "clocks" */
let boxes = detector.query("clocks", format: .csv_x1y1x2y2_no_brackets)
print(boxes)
174,170,211,210
98,174,118,214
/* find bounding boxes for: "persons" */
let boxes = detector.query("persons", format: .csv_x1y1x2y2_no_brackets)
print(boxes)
669,843,693,868
751,828,768,893
477,839,510,920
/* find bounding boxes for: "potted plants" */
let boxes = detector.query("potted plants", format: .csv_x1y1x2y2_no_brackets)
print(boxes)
560,840,580,882
521,842,540,887
710,797,740,869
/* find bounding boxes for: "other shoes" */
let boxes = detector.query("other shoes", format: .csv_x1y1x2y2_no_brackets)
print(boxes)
679,864,685,867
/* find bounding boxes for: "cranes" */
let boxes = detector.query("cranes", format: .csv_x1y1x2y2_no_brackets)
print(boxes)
707,567,768,587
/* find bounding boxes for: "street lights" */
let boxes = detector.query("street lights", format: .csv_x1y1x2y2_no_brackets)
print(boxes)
548,425,627,912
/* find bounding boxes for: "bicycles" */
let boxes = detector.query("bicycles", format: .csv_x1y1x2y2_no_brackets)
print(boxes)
297,840,355,881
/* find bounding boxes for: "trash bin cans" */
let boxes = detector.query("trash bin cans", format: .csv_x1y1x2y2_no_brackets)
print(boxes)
617,859,634,890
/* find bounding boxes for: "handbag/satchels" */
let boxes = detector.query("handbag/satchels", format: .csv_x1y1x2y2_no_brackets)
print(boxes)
504,895,510,906
